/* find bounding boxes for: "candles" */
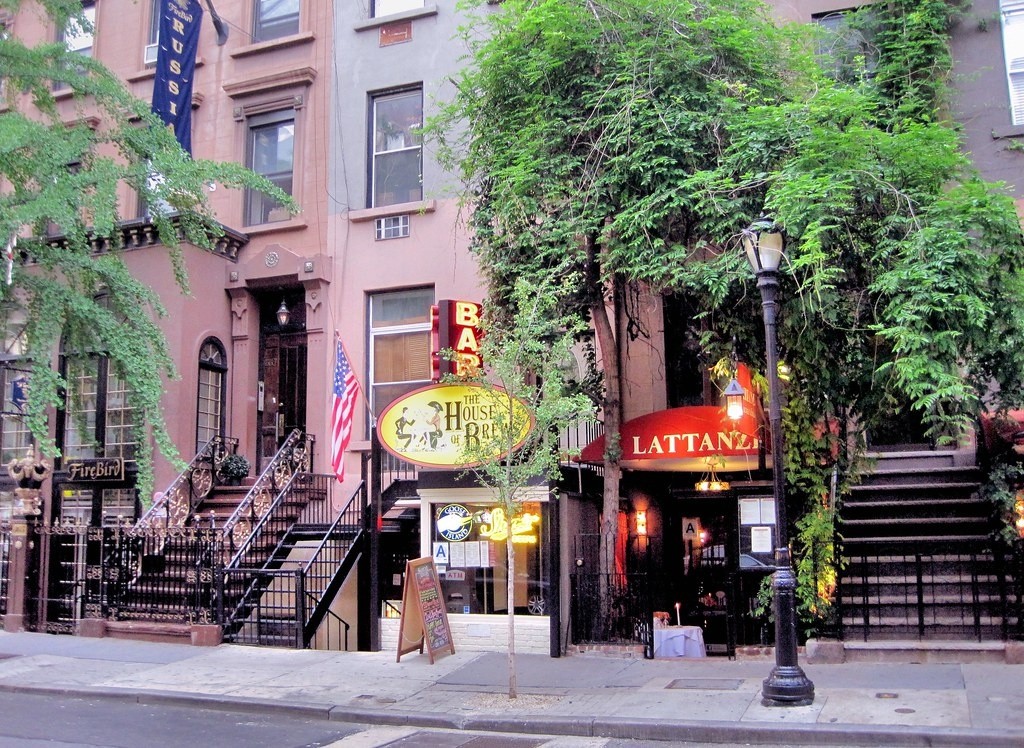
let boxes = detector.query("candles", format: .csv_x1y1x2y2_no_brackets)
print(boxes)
676,602,680,624
708,591,713,609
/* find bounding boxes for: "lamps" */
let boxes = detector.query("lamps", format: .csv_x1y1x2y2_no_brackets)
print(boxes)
696,457,731,492
630,497,652,537
723,336,744,421
275,293,304,326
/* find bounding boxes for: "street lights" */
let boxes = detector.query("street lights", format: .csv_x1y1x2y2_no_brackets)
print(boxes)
739,213,815,709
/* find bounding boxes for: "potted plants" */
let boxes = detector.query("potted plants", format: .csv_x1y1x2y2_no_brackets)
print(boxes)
381,118,406,152
220,455,252,486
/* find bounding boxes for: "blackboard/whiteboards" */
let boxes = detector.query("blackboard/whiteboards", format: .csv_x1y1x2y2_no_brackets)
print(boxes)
397,555,454,657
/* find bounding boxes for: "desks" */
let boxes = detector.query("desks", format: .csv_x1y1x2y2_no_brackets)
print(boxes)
641,626,705,658
674,610,739,660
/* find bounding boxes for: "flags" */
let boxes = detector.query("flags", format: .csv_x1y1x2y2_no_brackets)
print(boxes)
330,342,359,482
